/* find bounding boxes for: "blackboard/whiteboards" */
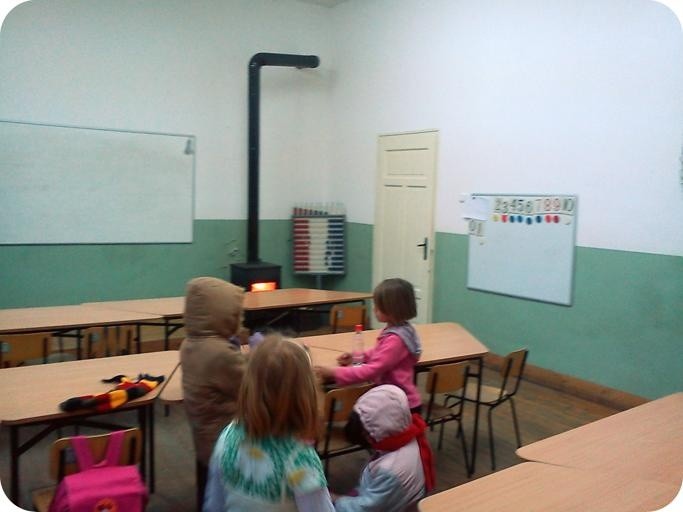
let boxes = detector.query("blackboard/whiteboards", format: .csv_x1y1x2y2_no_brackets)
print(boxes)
465,193,578,307
0,119,196,246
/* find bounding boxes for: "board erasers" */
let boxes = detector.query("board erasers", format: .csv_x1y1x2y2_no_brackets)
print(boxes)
185,141,192,154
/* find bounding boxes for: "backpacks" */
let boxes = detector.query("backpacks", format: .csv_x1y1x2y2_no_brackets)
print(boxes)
48,430,148,512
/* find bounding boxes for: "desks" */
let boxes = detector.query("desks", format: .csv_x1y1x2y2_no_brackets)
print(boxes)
416,391,683,512
296,321,491,476
0,338,345,495
84,289,375,417
1,305,166,367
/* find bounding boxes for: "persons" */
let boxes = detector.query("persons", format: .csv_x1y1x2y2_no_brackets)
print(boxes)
328,384,428,512
201,338,335,512
181,277,264,467
312,274,425,422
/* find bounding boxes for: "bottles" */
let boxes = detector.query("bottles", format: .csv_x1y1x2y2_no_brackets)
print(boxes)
351,324,363,369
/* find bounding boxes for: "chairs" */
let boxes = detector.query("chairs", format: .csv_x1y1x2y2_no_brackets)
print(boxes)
438,346,527,470
29,425,141,512
409,359,470,477
2,331,54,369
327,303,367,334
79,324,138,357
316,380,388,482
